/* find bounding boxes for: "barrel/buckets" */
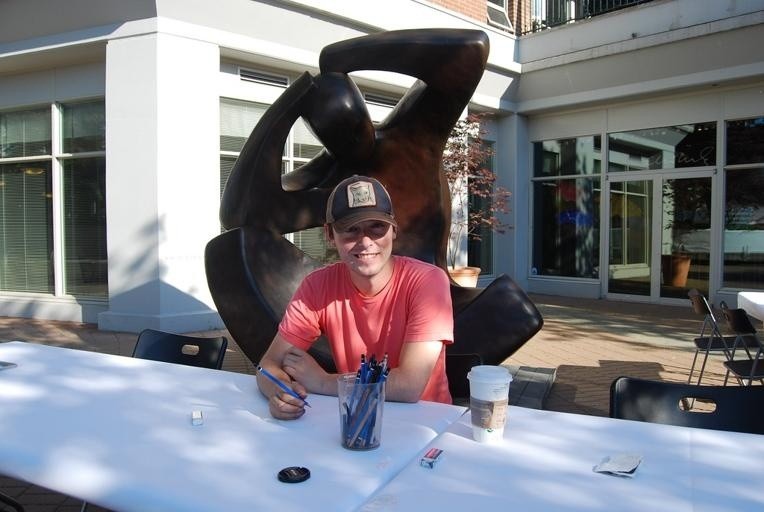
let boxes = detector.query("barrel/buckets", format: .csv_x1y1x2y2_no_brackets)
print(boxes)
662,244,692,287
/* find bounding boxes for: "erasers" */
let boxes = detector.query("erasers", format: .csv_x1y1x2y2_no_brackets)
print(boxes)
192,410,204,426
420,447,444,468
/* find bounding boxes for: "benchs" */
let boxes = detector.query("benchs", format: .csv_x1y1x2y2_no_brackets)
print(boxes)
689,288,763,388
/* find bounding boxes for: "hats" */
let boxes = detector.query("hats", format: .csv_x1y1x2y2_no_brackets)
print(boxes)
326,175,397,233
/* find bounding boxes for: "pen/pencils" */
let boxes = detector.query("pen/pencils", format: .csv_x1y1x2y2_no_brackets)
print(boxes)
342,351,391,449
258,366,312,409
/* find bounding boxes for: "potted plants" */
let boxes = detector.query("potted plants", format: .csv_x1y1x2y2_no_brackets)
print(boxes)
660,176,710,286
439,111,514,289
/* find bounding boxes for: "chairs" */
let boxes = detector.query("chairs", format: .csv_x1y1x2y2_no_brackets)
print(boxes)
130,329,228,369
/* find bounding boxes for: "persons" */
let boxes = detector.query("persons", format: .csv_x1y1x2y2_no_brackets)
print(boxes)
250,176,454,424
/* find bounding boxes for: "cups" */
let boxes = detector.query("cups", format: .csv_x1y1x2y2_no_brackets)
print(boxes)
467,363,513,446
335,371,385,451
